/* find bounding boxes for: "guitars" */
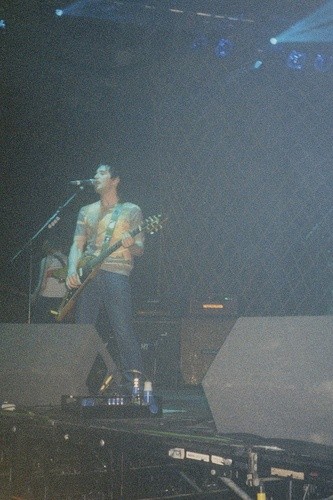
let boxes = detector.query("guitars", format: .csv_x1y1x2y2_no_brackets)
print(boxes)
49,210,170,321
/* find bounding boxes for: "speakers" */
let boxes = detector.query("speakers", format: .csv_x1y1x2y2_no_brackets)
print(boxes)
0,323,115,407
201,318,332,447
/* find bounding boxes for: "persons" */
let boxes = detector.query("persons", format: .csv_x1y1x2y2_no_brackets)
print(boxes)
30,237,68,324
65,159,145,393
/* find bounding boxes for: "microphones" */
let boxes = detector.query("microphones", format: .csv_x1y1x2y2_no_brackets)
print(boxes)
69,179,95,186
